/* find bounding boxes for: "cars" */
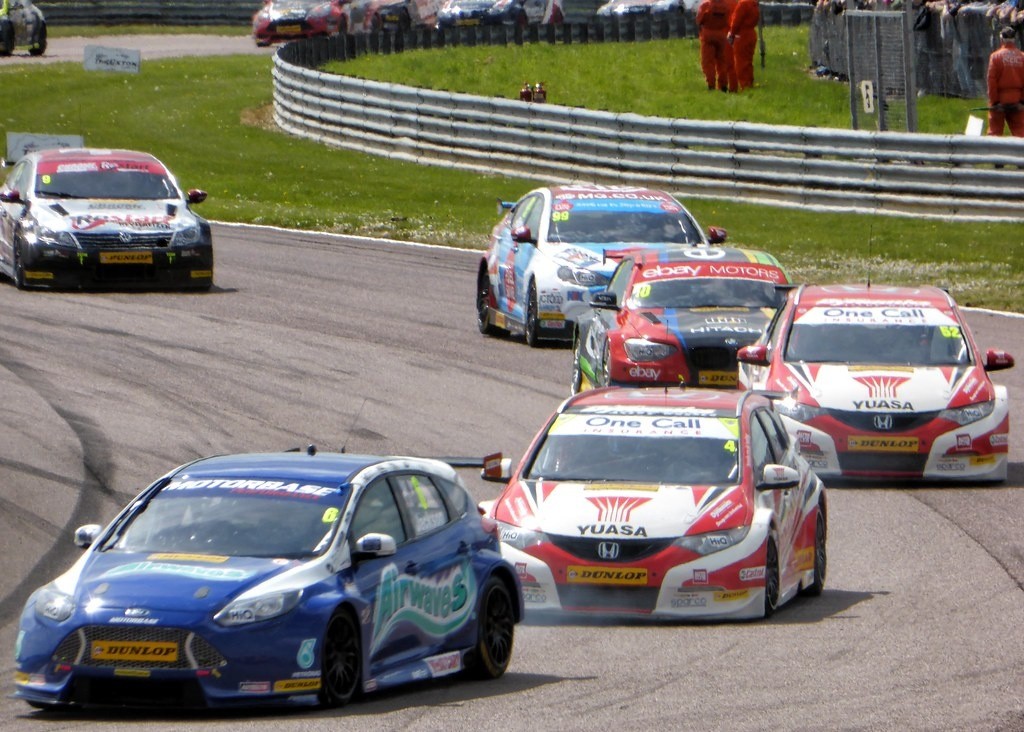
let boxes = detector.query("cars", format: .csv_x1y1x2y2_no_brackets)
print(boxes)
436,1,564,36
252,0,381,48
568,245,794,394
10,448,523,715
732,281,1016,487
474,185,728,346
596,0,706,21
378,0,448,34
473,383,831,625
0,0,48,57
0,147,214,292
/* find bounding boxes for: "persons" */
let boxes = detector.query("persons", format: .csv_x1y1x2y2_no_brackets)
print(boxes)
985,25,1024,171
696,0,737,92
716,0,743,94
725,0,761,91
807,0,1024,100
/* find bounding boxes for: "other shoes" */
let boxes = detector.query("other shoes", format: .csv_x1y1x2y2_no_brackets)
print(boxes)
915,88,960,100
808,61,850,82
708,84,727,93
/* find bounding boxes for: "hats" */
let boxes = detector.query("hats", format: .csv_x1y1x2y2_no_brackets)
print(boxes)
1002,27,1016,39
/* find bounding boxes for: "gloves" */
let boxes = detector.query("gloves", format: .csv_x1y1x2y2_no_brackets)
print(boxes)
726,31,735,44
